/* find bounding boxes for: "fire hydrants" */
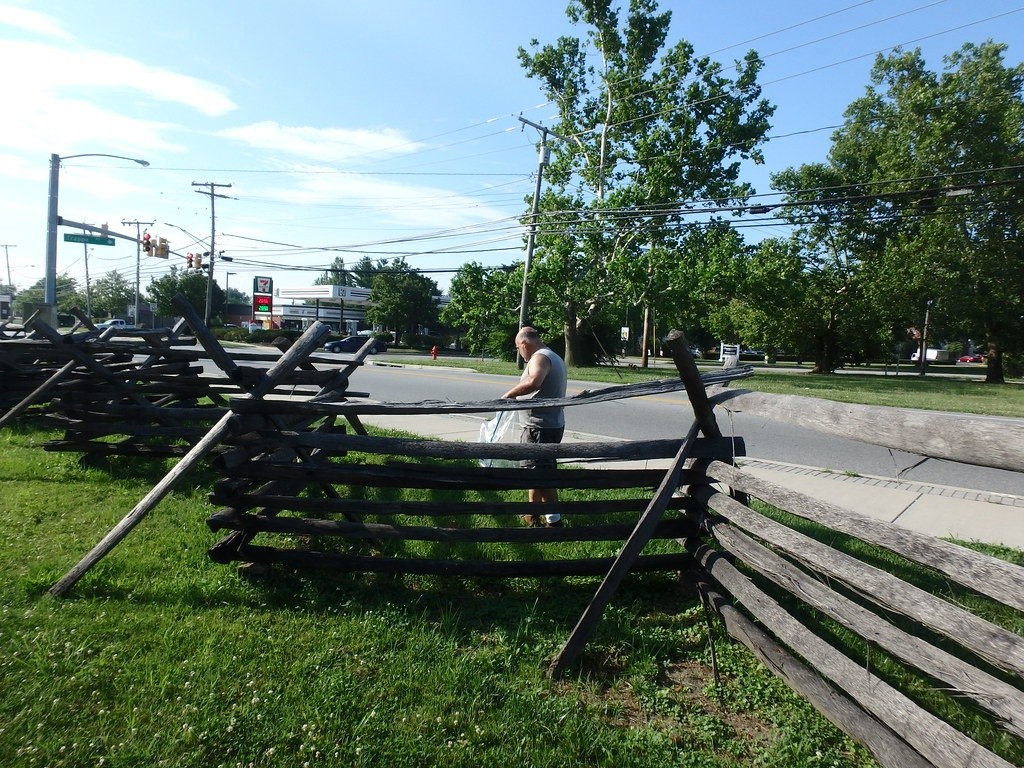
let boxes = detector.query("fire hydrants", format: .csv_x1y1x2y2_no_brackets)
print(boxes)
431,345,439,360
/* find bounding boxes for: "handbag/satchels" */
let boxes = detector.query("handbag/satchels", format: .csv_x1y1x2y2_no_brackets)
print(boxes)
477,400,530,468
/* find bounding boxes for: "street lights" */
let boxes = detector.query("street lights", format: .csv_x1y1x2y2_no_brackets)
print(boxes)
164,223,212,328
45,153,150,304
226,272,237,322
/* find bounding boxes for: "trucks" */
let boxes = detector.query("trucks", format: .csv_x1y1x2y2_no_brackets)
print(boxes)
911,347,949,364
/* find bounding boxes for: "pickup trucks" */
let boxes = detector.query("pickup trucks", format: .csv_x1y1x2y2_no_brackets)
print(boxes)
94,319,135,331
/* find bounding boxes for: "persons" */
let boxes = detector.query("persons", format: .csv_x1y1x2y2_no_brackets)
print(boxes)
502,326,567,529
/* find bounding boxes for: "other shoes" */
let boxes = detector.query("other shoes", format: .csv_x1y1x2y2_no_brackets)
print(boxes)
519,514,539,527
546,519,565,528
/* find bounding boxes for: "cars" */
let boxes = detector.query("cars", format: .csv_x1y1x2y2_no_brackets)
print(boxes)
958,354,985,363
324,336,387,355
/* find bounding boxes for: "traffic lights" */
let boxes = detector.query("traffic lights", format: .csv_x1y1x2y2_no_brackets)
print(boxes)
159,237,167,255
143,233,150,253
195,254,201,268
187,253,193,268
